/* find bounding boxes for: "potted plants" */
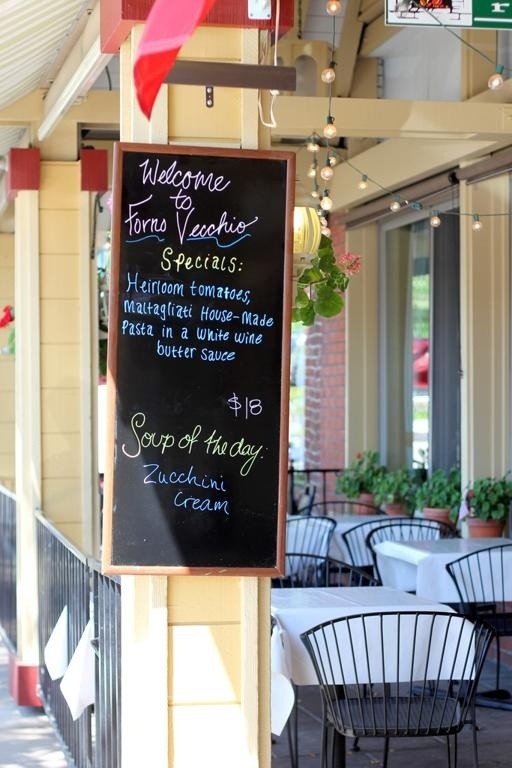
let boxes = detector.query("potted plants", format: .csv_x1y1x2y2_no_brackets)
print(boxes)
370,464,416,518
464,468,511,540
334,449,382,517
413,466,462,531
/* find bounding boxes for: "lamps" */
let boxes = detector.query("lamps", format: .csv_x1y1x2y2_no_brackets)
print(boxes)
293,197,322,256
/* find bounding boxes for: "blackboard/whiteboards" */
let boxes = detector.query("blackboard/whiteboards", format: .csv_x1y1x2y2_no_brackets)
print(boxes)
101,141,297,578
